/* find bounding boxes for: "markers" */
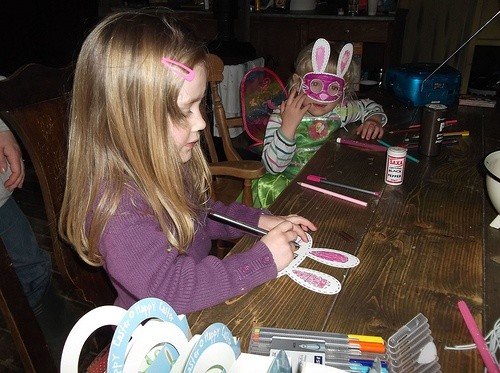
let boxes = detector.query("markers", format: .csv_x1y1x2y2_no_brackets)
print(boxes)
336,136,389,152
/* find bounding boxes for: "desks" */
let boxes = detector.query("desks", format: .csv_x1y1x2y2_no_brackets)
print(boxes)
84,99,500,373
110,4,239,42
247,7,409,85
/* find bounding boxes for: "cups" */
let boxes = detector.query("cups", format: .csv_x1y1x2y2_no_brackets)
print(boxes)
348,0,359,14
368,0,377,16
338,8,344,16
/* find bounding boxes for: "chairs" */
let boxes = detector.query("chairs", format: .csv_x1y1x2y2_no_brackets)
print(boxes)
0,16,276,373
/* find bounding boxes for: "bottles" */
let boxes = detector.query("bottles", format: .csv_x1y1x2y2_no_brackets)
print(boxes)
384,147,406,186
483,150,500,214
418,101,453,156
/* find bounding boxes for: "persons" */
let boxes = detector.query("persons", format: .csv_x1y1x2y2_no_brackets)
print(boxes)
236,41,388,209
58,9,317,334
0,119,91,356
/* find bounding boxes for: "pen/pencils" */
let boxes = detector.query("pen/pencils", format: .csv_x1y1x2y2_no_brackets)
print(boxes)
249,359,387,373
375,139,419,163
296,181,369,206
306,176,378,195
206,212,301,248
252,329,385,351
390,120,471,147
